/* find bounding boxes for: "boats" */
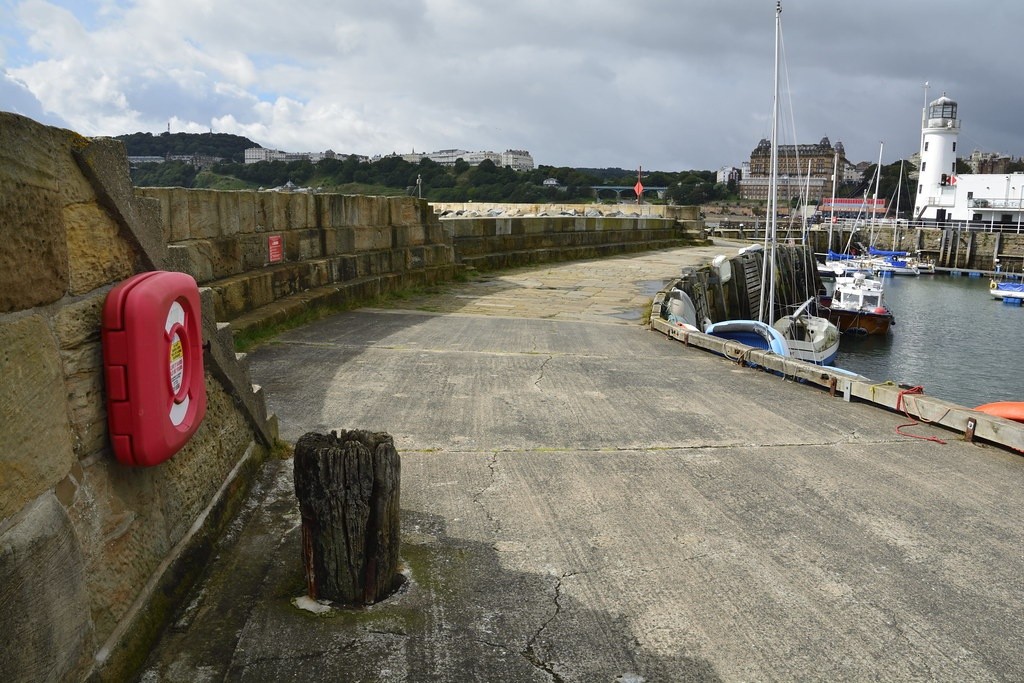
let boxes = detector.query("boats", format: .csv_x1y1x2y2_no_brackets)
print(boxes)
824,265,897,339
701,316,791,361
989,280,1024,304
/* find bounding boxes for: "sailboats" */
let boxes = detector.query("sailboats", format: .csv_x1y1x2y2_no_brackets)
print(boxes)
754,0,844,366
829,137,941,276
